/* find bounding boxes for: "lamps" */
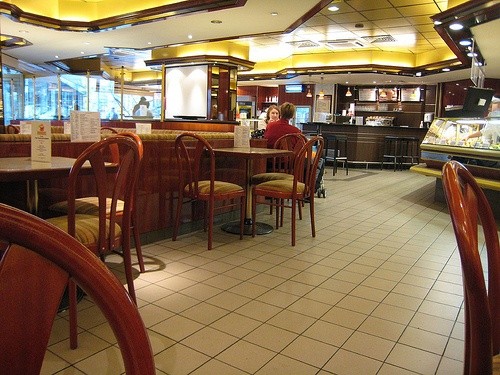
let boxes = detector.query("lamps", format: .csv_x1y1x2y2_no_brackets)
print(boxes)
345,72,352,97
380,74,386,97
306,73,312,97
319,74,324,100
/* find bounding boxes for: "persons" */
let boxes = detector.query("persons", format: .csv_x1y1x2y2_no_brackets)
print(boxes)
132,97,146,116
135,101,152,116
106,107,118,120
263,102,301,151
463,94,500,144
258,105,281,130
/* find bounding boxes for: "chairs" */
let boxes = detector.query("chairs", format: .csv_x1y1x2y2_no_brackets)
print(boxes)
441,160,500,375
170,133,326,249
0,124,156,375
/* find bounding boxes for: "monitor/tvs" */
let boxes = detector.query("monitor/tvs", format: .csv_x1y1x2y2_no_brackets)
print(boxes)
285,84,302,93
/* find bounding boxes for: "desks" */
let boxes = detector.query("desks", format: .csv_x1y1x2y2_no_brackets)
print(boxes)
206,146,293,235
0,156,118,315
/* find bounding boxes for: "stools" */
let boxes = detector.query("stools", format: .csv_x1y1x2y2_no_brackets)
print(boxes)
380,136,420,172
304,131,349,176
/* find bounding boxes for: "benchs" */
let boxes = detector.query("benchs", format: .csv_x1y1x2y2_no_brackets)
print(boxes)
0,127,259,247
